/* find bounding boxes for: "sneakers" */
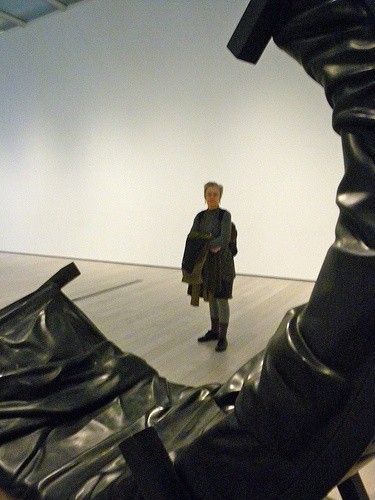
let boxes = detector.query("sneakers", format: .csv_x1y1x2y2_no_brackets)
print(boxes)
198,330,217,342
215,339,227,351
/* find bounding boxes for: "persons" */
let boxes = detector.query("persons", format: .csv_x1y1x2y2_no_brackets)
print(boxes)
191,182,236,352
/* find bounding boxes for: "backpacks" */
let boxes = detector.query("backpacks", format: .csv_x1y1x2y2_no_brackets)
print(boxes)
199,210,237,257
